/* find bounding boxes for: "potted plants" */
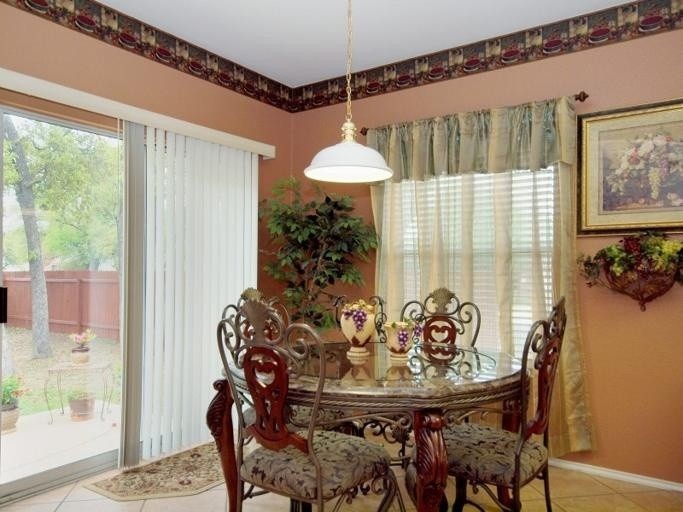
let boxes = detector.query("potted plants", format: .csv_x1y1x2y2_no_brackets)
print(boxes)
65,383,98,422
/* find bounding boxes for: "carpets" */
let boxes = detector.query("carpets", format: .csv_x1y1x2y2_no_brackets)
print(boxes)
82,437,253,503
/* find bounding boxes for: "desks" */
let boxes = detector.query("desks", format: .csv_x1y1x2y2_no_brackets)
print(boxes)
42,359,107,425
220,340,532,512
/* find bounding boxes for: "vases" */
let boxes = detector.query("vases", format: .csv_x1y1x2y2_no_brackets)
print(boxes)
71,348,90,365
0,404,21,435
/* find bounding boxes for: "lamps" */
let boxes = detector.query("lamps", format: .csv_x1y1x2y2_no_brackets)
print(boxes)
303,0,395,184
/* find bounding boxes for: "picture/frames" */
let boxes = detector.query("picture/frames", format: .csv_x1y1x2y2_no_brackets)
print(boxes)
576,96,683,238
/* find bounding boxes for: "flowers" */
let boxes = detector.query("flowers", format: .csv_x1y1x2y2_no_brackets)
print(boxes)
0,374,28,406
573,228,683,312
68,325,98,347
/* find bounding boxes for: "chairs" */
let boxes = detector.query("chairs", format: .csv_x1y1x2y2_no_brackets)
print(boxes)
410,294,568,512
399,287,483,348
216,299,405,510
